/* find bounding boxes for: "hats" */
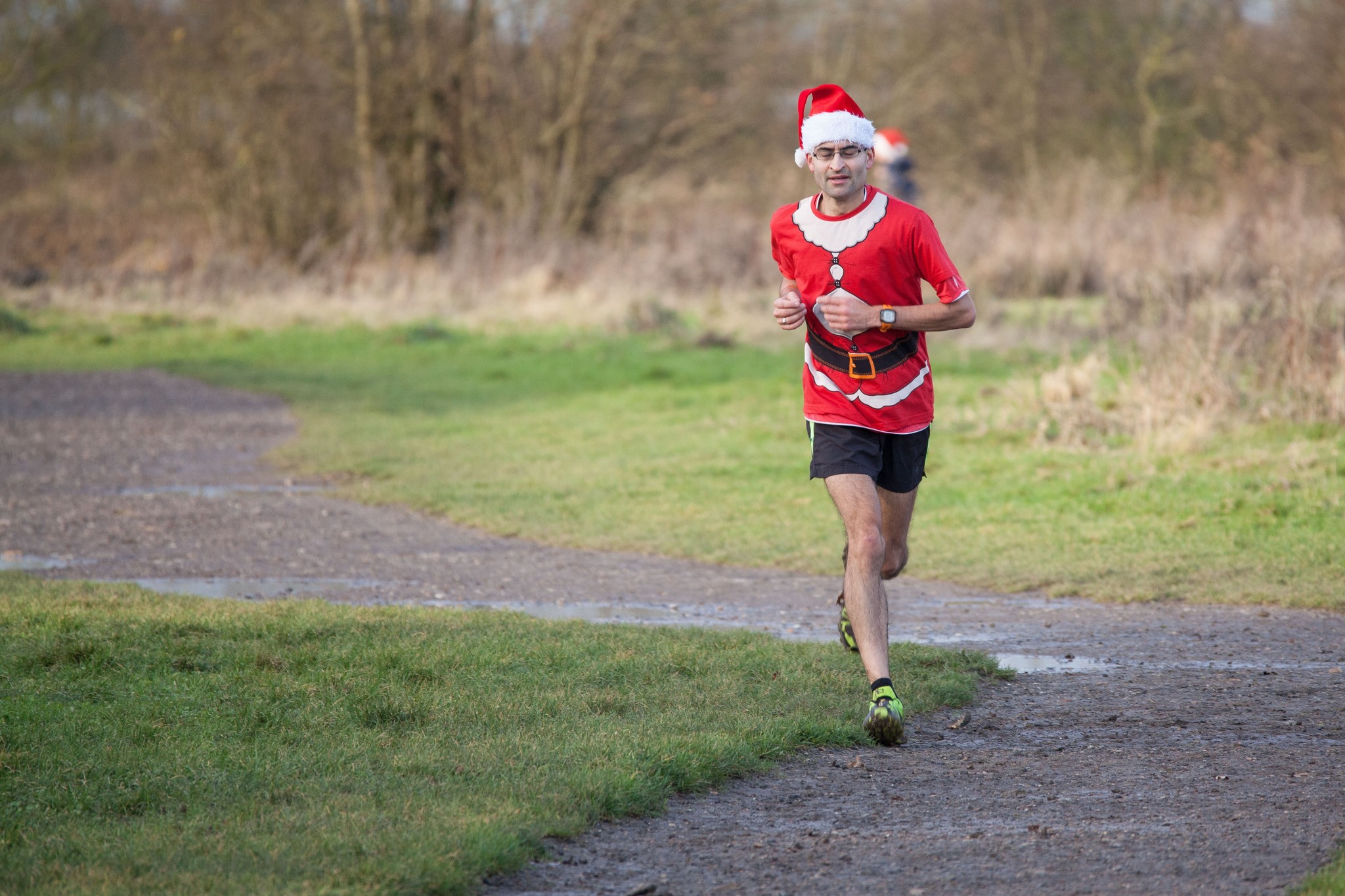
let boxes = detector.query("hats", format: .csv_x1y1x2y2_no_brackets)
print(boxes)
794,84,876,168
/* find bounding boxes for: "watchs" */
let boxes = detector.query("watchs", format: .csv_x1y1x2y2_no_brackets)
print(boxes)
879,305,896,333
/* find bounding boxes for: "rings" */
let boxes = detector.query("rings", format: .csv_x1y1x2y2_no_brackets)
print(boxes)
782,317,786,325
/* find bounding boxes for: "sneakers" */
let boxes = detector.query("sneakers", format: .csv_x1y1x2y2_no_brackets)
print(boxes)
837,588,859,652
864,685,905,745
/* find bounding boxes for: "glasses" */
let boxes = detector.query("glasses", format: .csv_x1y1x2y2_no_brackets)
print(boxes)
812,147,865,160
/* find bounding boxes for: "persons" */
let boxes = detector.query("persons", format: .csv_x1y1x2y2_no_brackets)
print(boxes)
770,85,978,750
884,149,915,204
